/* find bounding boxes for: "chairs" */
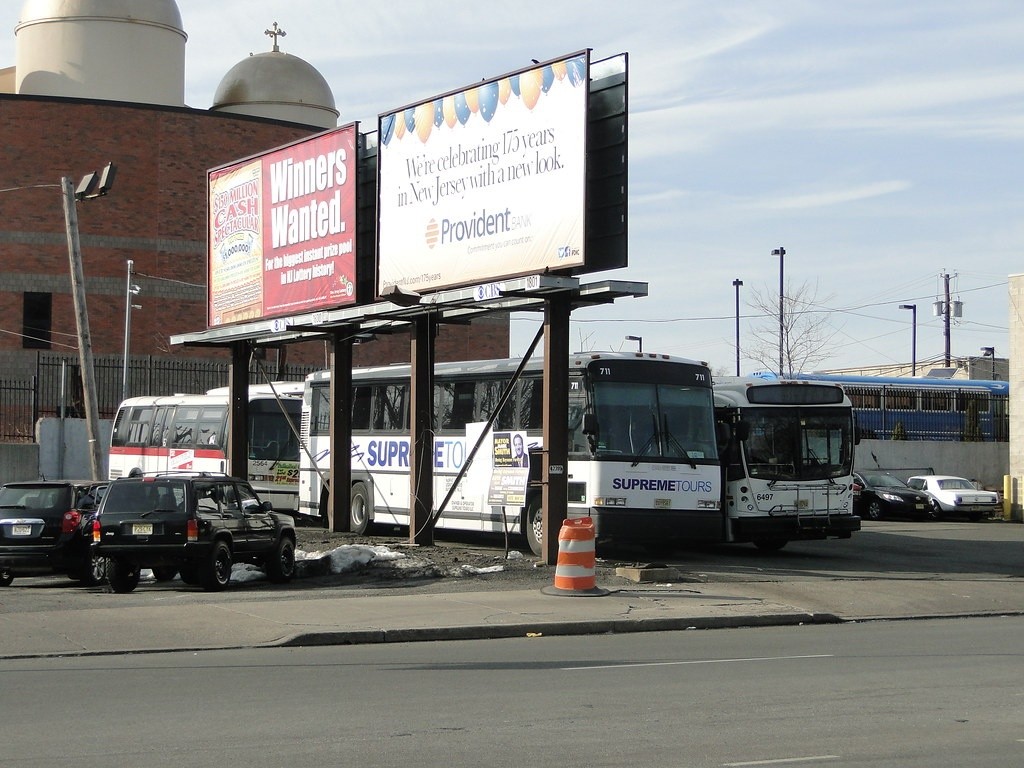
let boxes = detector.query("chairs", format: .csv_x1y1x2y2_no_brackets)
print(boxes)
159,494,178,510
854,477,881,490
165,432,300,461
26,493,59,508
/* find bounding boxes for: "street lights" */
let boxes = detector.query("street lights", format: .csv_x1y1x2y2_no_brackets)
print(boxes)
60,162,116,484
625,335,642,354
981,347,995,381
900,305,916,378
733,279,743,376
772,247,785,376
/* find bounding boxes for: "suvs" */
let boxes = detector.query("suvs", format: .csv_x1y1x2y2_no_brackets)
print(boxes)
89,472,297,592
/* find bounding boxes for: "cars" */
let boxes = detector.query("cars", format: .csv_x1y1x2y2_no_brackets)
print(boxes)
0,480,111,585
852,470,935,520
906,475,998,521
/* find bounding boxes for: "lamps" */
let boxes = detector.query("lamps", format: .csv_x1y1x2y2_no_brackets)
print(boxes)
379,285,429,307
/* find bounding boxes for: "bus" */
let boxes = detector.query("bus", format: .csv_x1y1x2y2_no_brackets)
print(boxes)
206,381,305,401
710,378,860,552
747,371,1009,441
109,394,302,508
298,354,722,555
